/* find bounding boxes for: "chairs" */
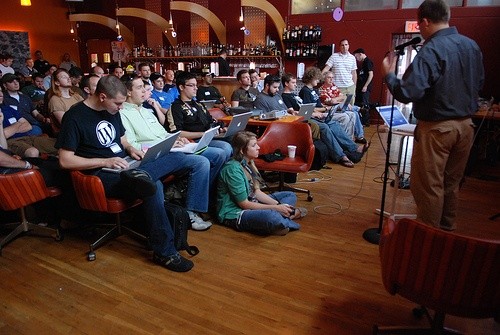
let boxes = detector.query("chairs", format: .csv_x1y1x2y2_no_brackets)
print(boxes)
253,122,315,202
0,168,147,262
377,215,499,334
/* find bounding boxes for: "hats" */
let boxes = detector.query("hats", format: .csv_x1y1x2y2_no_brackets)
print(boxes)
353,48,364,53
201,72,214,78
2,73,20,82
150,72,165,80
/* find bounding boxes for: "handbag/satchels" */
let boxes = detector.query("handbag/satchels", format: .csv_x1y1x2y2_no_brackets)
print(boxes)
166,203,200,256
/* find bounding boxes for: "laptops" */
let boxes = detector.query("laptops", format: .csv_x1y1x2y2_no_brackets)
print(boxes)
296,104,315,121
213,111,253,139
101,130,182,175
169,125,220,154
322,103,340,118
375,104,417,134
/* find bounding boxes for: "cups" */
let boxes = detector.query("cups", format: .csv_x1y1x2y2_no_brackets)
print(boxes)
288,145,297,158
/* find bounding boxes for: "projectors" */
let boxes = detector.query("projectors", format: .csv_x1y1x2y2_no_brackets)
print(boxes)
259,109,287,120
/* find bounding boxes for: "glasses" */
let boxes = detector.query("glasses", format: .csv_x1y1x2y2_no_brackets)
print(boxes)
186,84,197,87
125,70,138,84
245,90,252,98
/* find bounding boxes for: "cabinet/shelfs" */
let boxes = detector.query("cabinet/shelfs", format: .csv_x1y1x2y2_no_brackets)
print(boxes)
126,56,282,81
284,39,321,60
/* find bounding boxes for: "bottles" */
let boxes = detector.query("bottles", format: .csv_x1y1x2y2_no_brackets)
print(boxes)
132,24,321,77
291,207,307,216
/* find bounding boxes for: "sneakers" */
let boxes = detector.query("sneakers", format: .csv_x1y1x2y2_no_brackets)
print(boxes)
187,211,212,230
153,251,194,272
120,170,156,197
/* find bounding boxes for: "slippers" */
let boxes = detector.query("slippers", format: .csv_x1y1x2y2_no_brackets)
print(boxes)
362,142,371,158
340,160,355,167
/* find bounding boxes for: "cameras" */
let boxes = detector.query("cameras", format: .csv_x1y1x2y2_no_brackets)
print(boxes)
289,207,295,216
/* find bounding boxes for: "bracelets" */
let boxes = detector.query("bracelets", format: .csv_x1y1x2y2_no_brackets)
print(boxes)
277,202,281,205
32,165,34,170
25,161,31,169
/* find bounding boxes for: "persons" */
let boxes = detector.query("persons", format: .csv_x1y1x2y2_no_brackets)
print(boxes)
165,72,246,163
0,53,59,227
54,74,196,272
299,66,356,141
197,70,230,111
213,129,308,235
254,74,356,168
46,69,85,125
18,57,87,117
231,70,269,107
209,49,231,77
59,53,77,73
320,39,357,105
120,73,226,231
353,48,374,126
82,64,179,113
33,50,51,72
318,71,368,144
281,72,371,168
381,0,483,232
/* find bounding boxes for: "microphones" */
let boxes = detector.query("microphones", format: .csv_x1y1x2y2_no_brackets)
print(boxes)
395,37,421,50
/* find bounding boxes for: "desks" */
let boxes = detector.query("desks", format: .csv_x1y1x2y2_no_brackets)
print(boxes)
472,110,500,156
218,115,305,138
378,124,416,182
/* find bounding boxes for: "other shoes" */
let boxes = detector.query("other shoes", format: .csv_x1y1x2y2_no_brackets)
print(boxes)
296,208,308,218
274,228,289,234
355,138,367,144
